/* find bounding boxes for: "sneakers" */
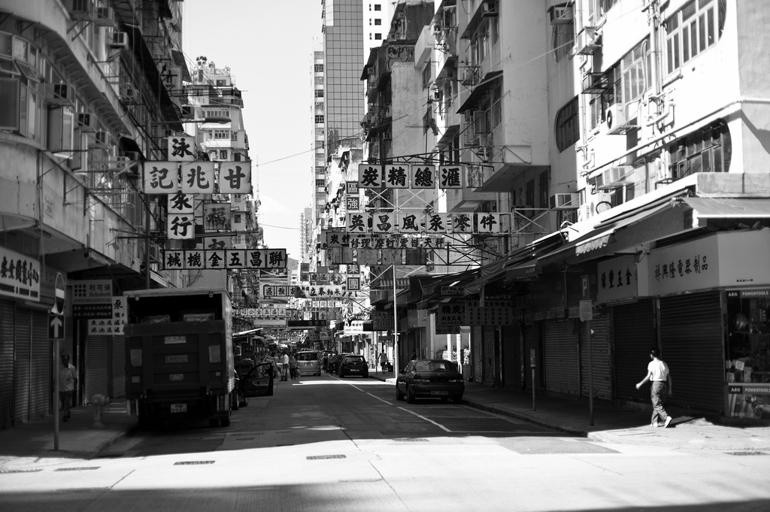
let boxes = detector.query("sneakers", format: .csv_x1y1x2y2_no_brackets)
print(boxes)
650,423,658,427
664,416,672,428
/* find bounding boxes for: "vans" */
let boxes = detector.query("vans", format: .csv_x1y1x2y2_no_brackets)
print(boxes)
294,350,321,376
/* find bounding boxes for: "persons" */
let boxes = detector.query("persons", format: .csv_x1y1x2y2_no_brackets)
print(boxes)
265,350,388,381
635,347,673,428
53,354,77,422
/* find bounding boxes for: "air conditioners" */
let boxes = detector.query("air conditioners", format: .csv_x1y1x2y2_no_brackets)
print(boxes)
97,7,130,52
55,83,111,146
576,30,595,50
550,193,579,211
605,103,626,131
550,7,572,25
603,166,633,187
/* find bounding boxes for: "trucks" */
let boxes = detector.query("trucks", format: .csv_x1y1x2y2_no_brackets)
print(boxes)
123,289,234,427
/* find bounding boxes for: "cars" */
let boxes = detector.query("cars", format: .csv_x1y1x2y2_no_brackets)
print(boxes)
338,354,369,378
232,360,273,410
396,359,465,404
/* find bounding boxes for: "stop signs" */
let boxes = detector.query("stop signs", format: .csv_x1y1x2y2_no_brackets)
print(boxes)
55,271,66,313
